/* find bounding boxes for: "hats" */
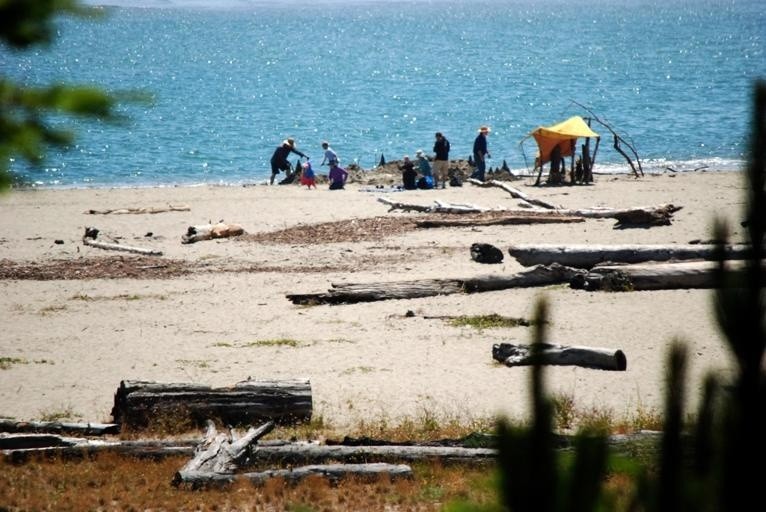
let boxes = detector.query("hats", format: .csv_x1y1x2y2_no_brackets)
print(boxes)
283,138,296,148
414,150,426,157
326,160,340,165
401,155,411,163
478,126,491,132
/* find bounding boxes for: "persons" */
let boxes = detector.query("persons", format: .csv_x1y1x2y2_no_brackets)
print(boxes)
404,164,418,189
470,126,492,181
397,156,415,171
327,160,348,190
320,142,337,170
415,149,432,176
298,162,318,189
269,137,310,186
433,132,450,188
